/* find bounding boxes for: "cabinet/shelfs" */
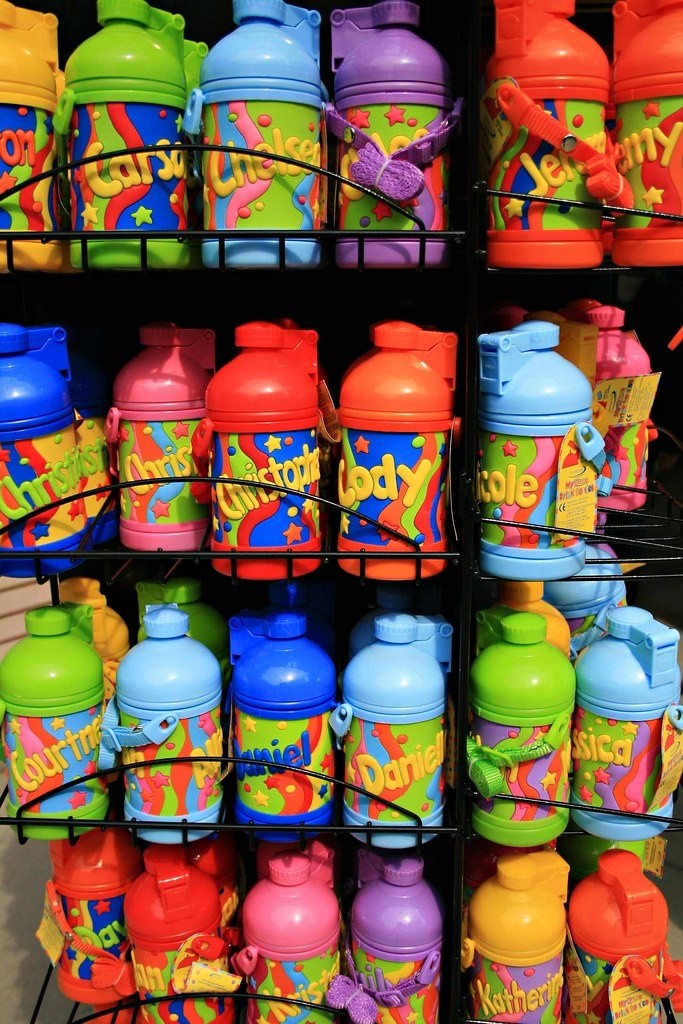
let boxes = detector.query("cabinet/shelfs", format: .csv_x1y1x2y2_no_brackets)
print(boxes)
0,2,683,1024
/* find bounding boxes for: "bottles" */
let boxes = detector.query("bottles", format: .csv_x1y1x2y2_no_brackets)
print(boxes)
38,805,444,1024
0,321,121,581
0,0,70,270
203,320,327,581
183,0,330,268
61,0,209,267
0,575,452,850
470,509,683,841
321,0,466,266
478,298,658,582
476,0,683,267
461,831,683,1024
335,321,460,580
105,326,216,554
